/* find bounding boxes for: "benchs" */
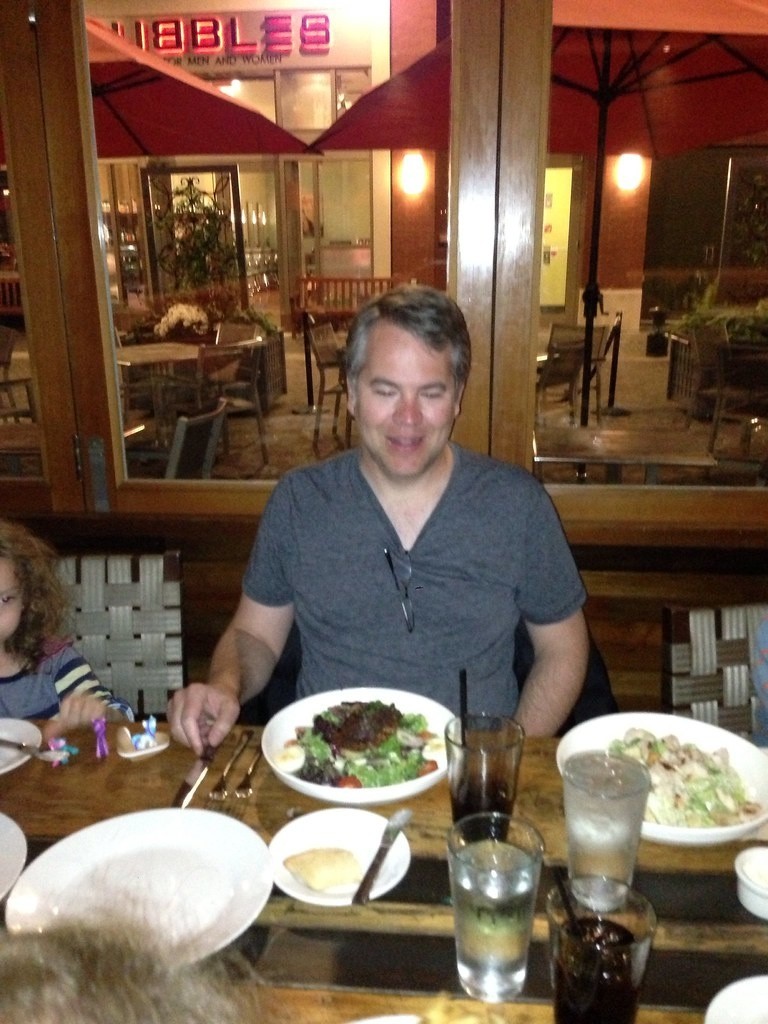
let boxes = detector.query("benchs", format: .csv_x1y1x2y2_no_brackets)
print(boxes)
291,278,393,341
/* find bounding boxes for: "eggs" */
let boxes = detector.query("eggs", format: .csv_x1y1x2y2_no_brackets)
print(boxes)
274,746,304,773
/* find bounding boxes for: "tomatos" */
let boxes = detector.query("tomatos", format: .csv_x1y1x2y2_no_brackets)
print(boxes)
336,777,362,788
419,761,438,776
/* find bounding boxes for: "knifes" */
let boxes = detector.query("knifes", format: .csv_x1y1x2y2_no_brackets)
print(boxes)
352,810,412,905
172,744,219,809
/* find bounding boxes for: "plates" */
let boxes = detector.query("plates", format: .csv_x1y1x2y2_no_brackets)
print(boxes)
0,813,27,900
0,718,42,774
269,808,411,907
5,808,273,961
556,712,768,844
261,687,457,803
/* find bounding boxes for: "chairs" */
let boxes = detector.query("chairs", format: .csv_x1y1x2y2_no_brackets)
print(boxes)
534,339,585,427
0,321,19,425
675,325,768,458
164,393,228,482
0,237,23,319
153,342,273,469
208,320,260,433
545,324,610,428
0,374,35,426
652,599,768,736
40,550,185,724
304,319,356,453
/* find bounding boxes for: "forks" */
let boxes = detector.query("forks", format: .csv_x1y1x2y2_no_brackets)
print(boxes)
204,729,254,812
227,743,262,820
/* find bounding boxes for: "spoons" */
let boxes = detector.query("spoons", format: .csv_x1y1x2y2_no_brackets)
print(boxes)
0,738,70,762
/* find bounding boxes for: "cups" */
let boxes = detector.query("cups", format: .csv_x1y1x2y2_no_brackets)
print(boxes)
545,877,656,1024
562,750,651,889
448,813,545,1004
445,714,526,824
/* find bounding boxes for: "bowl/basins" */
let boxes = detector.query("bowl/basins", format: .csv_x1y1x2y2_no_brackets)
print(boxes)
118,731,170,761
735,847,768,920
704,976,768,1023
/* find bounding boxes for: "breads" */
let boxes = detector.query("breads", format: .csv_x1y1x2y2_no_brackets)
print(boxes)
283,849,355,890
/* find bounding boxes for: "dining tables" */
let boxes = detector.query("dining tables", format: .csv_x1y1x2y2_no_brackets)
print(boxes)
0,722,768,1024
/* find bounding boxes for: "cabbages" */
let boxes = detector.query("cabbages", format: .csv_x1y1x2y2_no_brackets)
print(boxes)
612,728,746,827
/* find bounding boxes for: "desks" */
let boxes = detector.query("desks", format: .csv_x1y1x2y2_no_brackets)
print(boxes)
117,339,206,415
0,417,160,474
532,424,724,484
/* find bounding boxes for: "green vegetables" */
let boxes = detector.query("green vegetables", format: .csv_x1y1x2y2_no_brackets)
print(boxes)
300,713,428,786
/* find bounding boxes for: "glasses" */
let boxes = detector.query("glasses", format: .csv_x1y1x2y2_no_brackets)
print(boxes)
384,547,414,633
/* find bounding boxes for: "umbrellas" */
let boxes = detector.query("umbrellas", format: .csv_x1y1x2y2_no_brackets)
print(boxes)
0,15,326,168
301,0,768,484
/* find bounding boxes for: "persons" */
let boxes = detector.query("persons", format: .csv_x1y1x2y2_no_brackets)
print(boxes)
0,515,134,741
166,284,593,756
0,900,255,1024
